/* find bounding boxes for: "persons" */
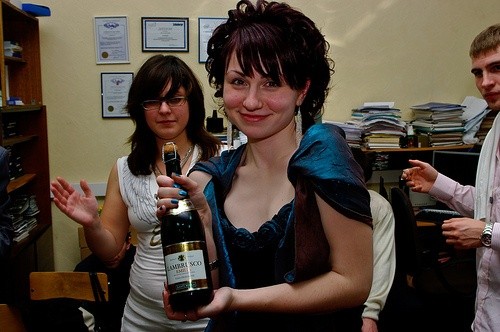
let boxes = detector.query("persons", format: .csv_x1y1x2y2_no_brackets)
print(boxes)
156,0,373,332
50,54,234,332
351,147,396,332
402,22,500,332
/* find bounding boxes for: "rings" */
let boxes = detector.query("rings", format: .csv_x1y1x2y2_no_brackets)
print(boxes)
182,312,188,322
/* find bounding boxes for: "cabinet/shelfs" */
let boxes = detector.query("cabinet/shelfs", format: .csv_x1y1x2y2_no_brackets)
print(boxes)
0,0,43,108
0,225,54,332
0,105,53,263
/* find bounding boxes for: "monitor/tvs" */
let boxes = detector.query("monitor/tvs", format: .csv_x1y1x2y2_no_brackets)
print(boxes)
432,151,480,200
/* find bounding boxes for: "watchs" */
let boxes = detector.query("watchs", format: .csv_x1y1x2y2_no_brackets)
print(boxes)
481,222,494,248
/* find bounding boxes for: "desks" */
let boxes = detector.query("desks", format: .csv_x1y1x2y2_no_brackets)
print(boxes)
416,217,440,227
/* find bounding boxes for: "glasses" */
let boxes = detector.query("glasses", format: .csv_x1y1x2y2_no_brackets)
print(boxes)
143,96,187,111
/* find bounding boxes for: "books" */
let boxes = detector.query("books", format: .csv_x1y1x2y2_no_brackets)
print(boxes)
4,40,26,57
211,122,248,158
322,102,407,149
0,115,24,183
1,188,41,242
409,95,500,148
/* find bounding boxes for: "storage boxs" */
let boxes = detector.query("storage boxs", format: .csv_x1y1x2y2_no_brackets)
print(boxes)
80,248,93,263
78,225,141,247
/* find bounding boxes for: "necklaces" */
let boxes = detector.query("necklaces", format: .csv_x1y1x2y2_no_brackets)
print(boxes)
154,144,195,177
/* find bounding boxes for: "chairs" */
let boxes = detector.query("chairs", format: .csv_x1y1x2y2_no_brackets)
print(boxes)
30,271,109,332
390,186,469,331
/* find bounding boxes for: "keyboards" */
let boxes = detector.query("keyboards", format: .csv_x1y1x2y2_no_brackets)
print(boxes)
414,210,464,224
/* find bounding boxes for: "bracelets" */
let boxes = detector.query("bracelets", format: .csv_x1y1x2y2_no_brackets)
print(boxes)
209,259,218,270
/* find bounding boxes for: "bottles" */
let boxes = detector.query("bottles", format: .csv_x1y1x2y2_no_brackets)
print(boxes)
160,142,214,314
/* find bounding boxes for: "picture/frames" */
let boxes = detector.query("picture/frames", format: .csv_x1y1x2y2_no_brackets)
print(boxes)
141,16,190,53
101,72,134,119
198,17,229,64
94,15,131,65
